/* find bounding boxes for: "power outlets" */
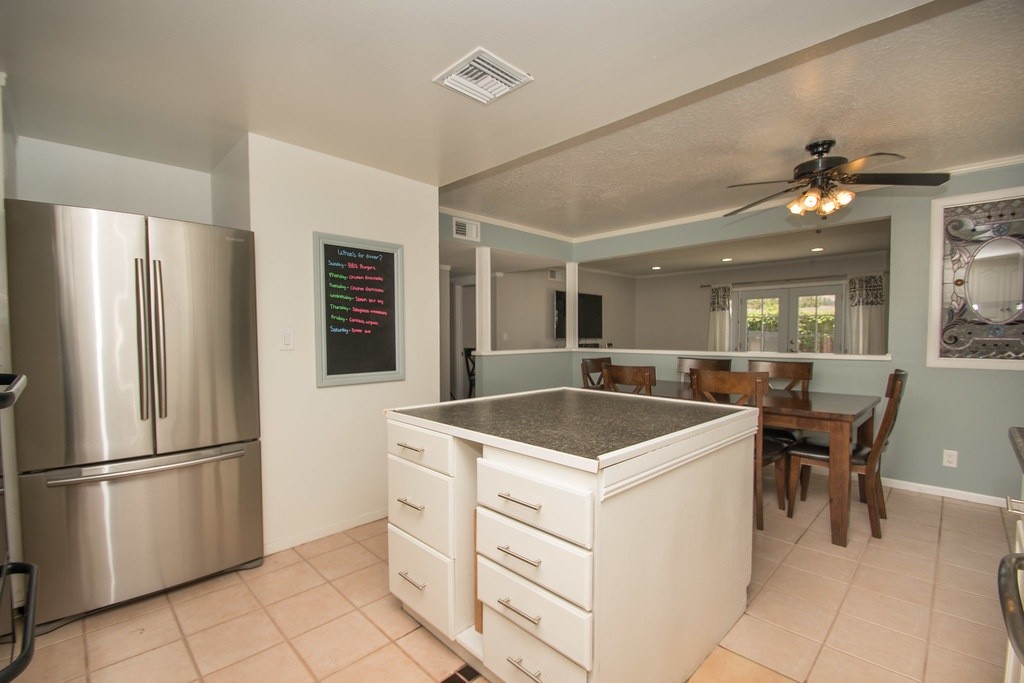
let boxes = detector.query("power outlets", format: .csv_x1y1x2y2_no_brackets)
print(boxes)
942,449,959,468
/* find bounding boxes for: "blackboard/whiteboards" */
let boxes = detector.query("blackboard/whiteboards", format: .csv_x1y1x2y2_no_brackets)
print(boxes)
311,230,406,389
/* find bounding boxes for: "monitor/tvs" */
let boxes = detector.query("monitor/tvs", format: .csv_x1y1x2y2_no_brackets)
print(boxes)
554,290,603,339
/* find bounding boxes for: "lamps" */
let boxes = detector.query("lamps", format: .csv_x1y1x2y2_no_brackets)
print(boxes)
785,179,856,216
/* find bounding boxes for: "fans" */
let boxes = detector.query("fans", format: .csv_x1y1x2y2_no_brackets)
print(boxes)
722,139,951,218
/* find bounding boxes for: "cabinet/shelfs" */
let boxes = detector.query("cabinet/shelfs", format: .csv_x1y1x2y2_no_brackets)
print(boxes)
382,386,760,683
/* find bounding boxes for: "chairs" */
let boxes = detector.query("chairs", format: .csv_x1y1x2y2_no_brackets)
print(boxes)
689,367,796,530
787,368,908,538
748,359,813,501
601,362,656,396
676,357,733,383
580,357,612,387
464,348,476,398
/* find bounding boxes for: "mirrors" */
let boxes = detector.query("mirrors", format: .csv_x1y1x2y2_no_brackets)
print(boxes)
924,185,1024,371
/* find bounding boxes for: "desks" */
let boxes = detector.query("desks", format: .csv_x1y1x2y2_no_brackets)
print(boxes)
583,379,882,548
